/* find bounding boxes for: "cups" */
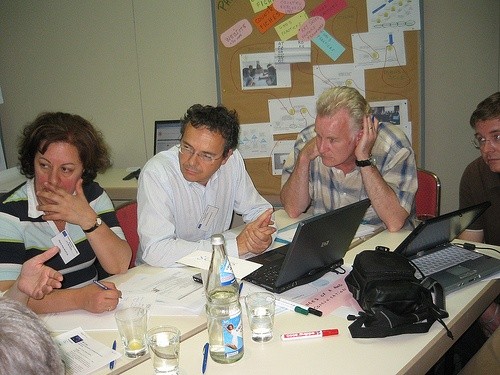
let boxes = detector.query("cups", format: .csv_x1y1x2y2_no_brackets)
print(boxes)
244,291,275,342
113,301,148,358
145,325,182,375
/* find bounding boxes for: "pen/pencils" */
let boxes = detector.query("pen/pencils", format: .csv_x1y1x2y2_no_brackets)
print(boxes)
372,115,374,123
202,343,209,373
110,340,117,370
93,280,122,298
239,282,243,294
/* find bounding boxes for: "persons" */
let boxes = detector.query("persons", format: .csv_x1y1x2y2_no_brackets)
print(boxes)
134,104,277,270
0,297,72,375
242,59,277,86
279,85,419,233
0,245,65,309
456,90,500,339
0,111,132,315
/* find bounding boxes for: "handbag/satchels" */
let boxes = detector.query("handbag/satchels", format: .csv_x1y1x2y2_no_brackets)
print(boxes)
344,245,453,341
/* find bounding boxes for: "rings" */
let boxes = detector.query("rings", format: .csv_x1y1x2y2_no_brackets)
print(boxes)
107,304,113,311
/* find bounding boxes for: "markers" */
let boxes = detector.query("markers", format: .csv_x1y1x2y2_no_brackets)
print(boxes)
281,329,339,341
279,298,323,317
266,297,308,315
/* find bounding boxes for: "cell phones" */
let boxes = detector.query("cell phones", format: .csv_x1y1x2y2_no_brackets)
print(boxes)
193,273,202,284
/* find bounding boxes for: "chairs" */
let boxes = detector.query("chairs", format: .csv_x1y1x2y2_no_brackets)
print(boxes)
115,199,139,270
415,167,441,220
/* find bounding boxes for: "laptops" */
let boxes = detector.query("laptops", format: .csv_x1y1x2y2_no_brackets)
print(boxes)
154,120,183,156
393,201,500,295
243,198,371,294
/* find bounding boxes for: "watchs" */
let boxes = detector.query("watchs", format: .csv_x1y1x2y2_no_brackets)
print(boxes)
82,215,103,233
353,154,378,167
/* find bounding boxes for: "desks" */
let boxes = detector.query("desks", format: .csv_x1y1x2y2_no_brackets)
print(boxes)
34,208,500,375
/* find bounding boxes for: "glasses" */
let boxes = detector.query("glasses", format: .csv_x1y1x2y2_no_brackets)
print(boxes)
471,135,500,149
175,140,225,164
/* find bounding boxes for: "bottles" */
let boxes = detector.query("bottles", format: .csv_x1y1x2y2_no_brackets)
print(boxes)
203,233,244,364
